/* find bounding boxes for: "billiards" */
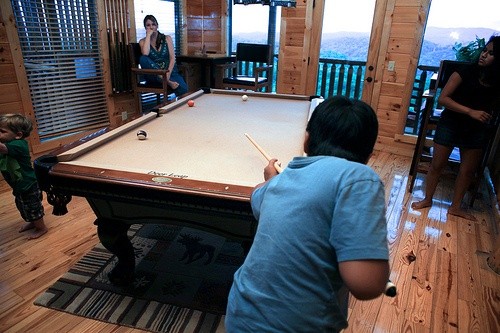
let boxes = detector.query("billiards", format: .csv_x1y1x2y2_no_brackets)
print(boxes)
136,130,147,140
241,94,248,101
187,100,194,107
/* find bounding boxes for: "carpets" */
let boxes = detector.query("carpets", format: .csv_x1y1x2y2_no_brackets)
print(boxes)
34,224,246,333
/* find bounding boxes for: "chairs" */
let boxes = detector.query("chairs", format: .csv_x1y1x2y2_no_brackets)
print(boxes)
408,60,500,206
221,43,273,92
129,42,189,112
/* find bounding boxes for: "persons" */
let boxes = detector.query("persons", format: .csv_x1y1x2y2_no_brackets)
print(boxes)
139,15,189,97
412,35,500,222
224,96,390,333
0,113,48,240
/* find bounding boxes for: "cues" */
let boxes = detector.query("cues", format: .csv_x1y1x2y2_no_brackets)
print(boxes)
244,133,282,174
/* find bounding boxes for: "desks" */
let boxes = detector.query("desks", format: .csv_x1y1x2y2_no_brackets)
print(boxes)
174,51,236,90
34,86,325,286
